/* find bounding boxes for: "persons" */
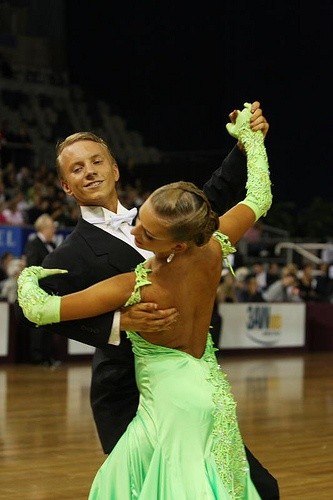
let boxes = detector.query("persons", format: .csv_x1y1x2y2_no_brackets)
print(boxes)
0,127,333,366
17,104,273,500
38,101,280,500
